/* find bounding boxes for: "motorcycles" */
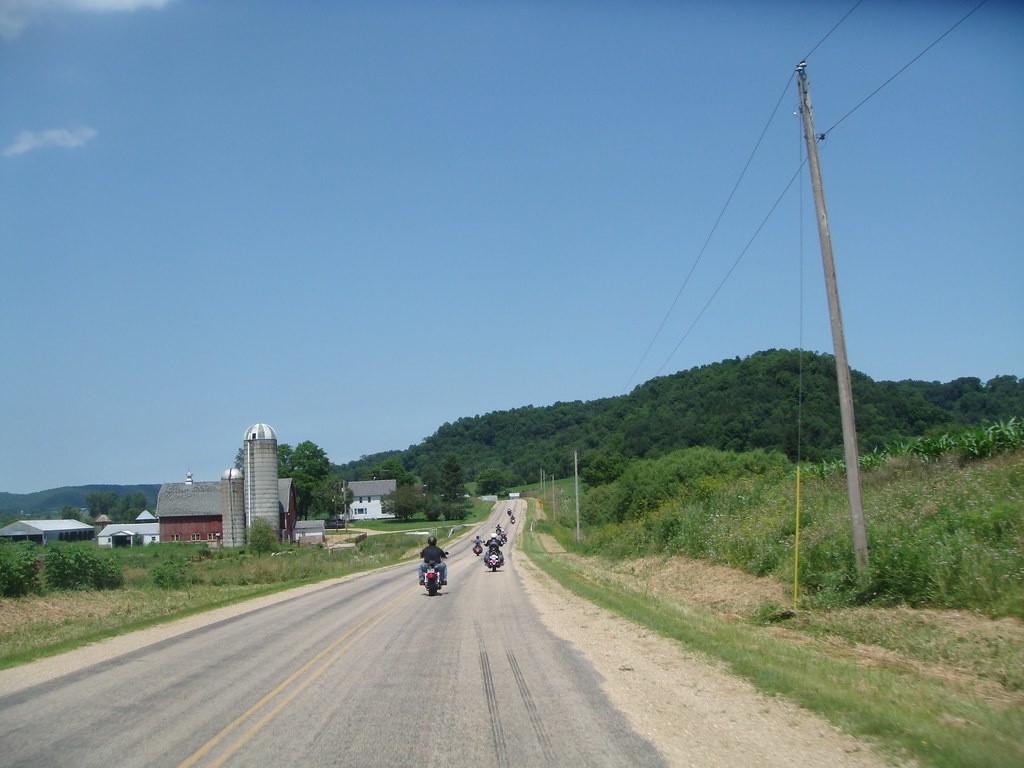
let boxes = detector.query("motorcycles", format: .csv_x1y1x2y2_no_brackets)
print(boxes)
507,510,511,516
511,518,515,524
471,540,486,556
502,531,508,544
484,543,504,572
420,550,449,596
496,527,502,535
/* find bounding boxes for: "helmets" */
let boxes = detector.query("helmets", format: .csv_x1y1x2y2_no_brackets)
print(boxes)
428,536,437,545
476,535,479,538
501,530,504,532
491,533,497,539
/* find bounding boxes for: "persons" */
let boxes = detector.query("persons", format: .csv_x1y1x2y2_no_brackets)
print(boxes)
496,524,501,529
507,508,511,514
485,533,503,555
500,530,507,542
511,515,514,519
419,536,447,583
473,536,482,545
